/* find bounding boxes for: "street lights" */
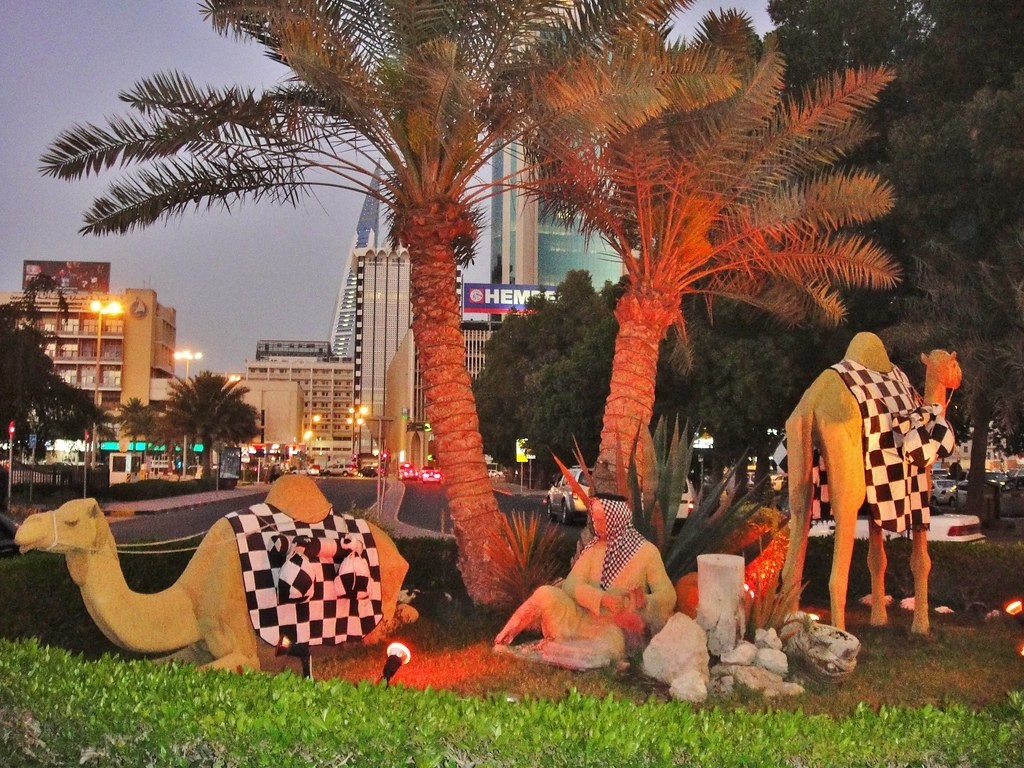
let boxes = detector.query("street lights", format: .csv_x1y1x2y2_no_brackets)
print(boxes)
86,295,127,470
172,348,204,479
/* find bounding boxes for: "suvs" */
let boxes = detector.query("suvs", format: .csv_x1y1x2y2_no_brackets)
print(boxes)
542,465,600,524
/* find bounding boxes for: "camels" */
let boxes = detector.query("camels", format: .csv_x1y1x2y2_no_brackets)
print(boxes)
14,475,419,675
783,333,963,634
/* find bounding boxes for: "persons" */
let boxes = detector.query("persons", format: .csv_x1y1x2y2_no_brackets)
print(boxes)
492,493,677,669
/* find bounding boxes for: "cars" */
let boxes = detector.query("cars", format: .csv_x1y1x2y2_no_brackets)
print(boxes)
275,451,509,488
728,452,1023,575
641,466,699,519
